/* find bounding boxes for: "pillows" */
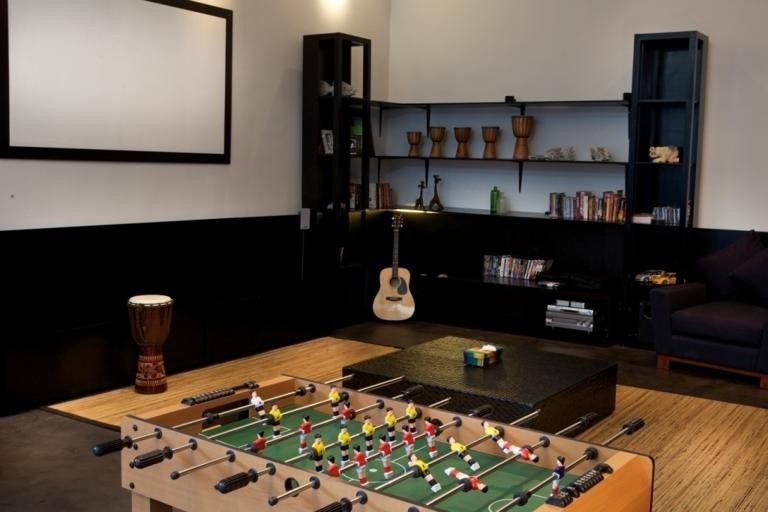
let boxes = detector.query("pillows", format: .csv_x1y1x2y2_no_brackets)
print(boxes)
695,231,763,300
727,247,768,305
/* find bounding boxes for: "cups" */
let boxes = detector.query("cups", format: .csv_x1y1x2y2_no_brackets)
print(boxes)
653,206,680,226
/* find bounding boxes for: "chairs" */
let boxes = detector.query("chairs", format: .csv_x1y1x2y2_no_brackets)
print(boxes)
649,231,768,391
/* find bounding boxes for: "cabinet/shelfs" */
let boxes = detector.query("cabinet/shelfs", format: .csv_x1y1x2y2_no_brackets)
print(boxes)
301,30,710,350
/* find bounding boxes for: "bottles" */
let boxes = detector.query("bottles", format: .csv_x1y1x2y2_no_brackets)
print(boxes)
497,192,506,215
491,185,499,214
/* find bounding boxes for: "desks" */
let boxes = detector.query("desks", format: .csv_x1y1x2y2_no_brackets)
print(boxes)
341,335,617,438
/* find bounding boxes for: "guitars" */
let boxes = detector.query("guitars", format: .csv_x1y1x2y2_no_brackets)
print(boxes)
373,213,416,321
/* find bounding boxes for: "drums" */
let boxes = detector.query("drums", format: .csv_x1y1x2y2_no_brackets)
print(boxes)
408,131,421,157
128,294,175,394
512,116,533,159
429,127,445,157
482,127,497,159
454,127,472,158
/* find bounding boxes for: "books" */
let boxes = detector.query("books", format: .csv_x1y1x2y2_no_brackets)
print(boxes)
346,181,394,208
481,253,553,281
631,205,680,225
548,187,627,223
